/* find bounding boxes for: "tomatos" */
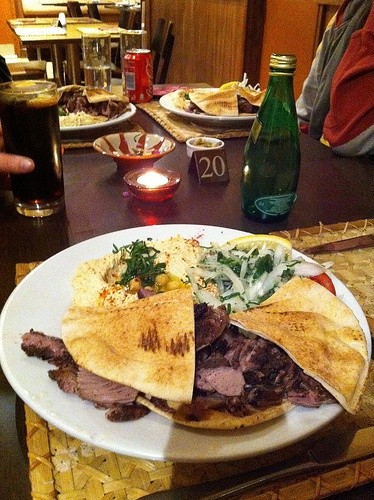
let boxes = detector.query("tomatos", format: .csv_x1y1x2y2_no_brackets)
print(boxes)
309,274,337,297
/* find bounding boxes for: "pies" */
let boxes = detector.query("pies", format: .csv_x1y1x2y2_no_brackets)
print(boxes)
188,89,239,117
236,86,267,107
56,84,84,91
227,275,370,414
134,394,292,429
83,86,129,103
61,289,195,404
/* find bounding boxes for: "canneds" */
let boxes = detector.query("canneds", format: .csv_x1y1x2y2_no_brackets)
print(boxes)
123,47,153,103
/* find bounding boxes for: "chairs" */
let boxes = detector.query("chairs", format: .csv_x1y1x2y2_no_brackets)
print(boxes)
0,0,176,85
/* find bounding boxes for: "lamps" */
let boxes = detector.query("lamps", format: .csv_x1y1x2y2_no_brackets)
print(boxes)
120,165,181,198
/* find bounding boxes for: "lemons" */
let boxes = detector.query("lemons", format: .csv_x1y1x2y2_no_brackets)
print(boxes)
219,81,239,91
220,234,292,256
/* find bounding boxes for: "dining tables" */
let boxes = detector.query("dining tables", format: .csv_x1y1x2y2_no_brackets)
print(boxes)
61,81,374,500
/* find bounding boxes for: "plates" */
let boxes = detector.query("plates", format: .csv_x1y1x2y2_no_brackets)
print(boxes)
60,103,137,135
0,224,372,466
159,87,256,132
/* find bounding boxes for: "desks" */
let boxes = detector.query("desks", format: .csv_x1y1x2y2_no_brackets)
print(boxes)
40,0,86,18
4,16,132,84
86,0,116,20
122,6,142,30
105,0,135,30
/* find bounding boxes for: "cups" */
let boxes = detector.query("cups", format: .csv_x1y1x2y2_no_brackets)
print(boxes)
81,32,112,93
0,80,66,218
119,29,148,97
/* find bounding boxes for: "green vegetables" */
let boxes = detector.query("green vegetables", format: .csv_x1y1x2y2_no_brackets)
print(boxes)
109,238,302,306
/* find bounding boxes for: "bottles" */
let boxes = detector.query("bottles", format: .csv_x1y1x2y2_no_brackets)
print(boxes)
241,52,301,224
57,11,66,34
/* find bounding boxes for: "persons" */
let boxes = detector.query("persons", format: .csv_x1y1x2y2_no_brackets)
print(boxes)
0,135,64,174
294,0,374,157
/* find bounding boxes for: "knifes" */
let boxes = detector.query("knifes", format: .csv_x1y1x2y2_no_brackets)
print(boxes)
302,234,374,255
136,426,374,500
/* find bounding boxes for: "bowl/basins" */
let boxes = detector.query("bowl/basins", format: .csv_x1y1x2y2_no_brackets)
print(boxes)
123,167,181,203
92,132,176,170
186,136,225,157
